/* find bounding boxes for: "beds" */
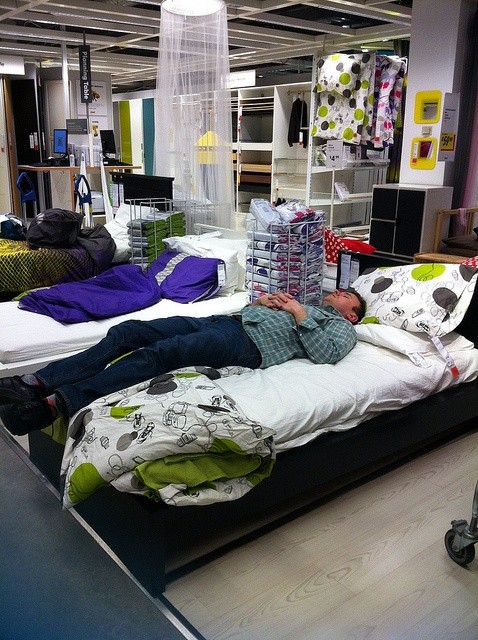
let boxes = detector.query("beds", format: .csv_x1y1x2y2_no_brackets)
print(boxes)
0,222,250,379
0,202,160,294
433,206,478,258
28,249,478,599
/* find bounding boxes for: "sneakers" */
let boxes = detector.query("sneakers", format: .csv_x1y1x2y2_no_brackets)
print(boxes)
0,374,43,401
0,398,54,435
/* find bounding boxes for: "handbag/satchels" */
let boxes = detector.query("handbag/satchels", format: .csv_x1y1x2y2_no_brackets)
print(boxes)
27,208,86,248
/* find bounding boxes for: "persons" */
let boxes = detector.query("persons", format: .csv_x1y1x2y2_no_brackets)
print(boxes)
0,286,365,435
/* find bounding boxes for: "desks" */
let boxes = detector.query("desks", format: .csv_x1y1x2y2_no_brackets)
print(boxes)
412,252,470,264
16,164,141,213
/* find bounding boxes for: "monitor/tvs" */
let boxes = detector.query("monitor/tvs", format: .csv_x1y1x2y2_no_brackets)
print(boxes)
53,128,68,160
100,129,119,161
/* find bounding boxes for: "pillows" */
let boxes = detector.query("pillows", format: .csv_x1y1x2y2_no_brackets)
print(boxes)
162,235,241,296
349,262,477,379
439,246,478,258
114,201,160,230
440,235,477,251
224,238,248,292
340,238,377,255
322,226,352,261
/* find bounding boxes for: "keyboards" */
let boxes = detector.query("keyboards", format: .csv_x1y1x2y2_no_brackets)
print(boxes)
28,163,50,168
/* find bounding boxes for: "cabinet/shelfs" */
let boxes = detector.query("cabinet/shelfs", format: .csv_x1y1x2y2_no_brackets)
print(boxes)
243,217,327,309
309,61,392,244
170,81,312,227
125,197,189,272
368,183,454,261
